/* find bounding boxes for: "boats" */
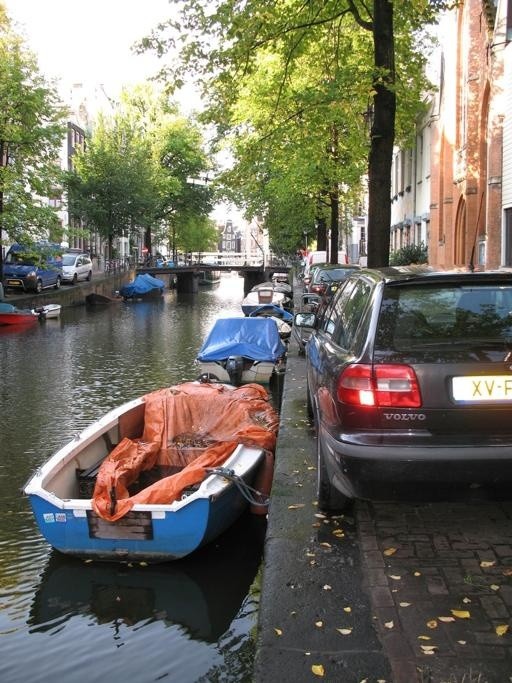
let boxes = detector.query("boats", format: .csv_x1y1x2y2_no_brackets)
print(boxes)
22,509,263,644
240,271,294,338
199,269,221,284
20,382,277,564
122,284,164,299
30,302,62,319
85,290,125,306
195,317,286,394
0,303,39,323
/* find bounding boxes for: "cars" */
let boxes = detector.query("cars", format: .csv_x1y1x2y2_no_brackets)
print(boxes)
291,248,358,358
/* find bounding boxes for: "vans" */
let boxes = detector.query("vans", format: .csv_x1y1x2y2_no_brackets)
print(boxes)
2,241,64,294
59,250,93,285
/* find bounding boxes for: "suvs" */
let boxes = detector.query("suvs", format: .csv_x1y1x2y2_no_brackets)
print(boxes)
294,263,512,514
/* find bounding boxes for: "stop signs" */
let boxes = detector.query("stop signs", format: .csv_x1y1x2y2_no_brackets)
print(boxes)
141,246,149,254
